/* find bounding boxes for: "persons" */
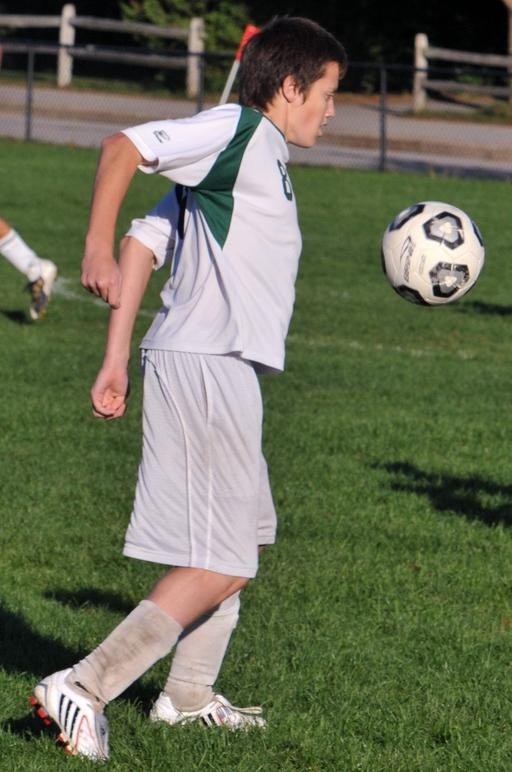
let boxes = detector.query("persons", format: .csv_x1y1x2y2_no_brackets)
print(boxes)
29,16,348,762
0,216,58,321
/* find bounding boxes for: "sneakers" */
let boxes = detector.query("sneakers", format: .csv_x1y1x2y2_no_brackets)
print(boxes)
30,664,112,763
146,686,266,736
23,255,60,323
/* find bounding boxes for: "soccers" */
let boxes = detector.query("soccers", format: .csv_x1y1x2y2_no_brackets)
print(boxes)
380,199,486,306
